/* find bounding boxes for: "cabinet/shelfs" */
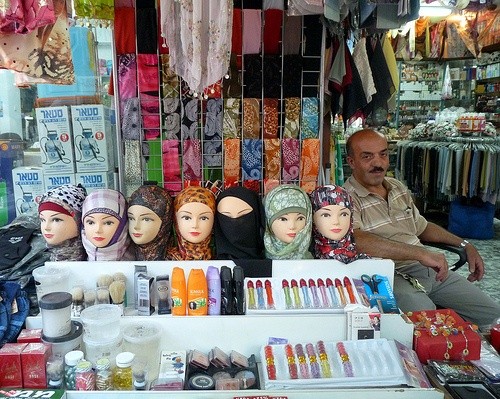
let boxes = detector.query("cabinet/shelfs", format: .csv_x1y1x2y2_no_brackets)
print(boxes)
383,58,500,136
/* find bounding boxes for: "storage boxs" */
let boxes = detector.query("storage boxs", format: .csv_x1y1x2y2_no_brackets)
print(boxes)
0,328,52,389
12,104,119,217
159,350,187,382
405,309,481,361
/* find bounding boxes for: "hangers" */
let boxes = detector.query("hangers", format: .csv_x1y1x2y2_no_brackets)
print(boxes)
397,137,500,155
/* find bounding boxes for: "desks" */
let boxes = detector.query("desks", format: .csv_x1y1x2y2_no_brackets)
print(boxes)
26,262,445,399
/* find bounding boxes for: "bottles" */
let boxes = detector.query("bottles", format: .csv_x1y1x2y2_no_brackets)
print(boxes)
113,352,135,391
95,358,113,391
75,360,95,390
64,351,85,390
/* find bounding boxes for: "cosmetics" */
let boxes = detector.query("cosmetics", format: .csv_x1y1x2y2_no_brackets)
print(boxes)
336,342,353,377
247,280,274,309
282,277,355,309
133,264,171,315
264,346,275,379
286,341,332,379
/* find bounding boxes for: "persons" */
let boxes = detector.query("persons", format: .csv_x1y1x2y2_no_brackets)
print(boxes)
81,189,131,261
126,185,174,261
264,183,313,259
342,129,500,327
213,186,272,277
38,183,87,260
166,186,215,261
309,185,359,264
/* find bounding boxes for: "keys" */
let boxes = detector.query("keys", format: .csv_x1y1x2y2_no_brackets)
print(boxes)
404,273,426,293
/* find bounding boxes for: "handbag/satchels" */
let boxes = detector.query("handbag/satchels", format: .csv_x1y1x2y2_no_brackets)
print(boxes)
447,196,496,240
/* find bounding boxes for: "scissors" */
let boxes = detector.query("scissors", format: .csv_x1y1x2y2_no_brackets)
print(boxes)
360,274,384,313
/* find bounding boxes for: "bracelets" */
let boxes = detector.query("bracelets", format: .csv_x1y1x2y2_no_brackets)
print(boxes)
459,240,470,248
